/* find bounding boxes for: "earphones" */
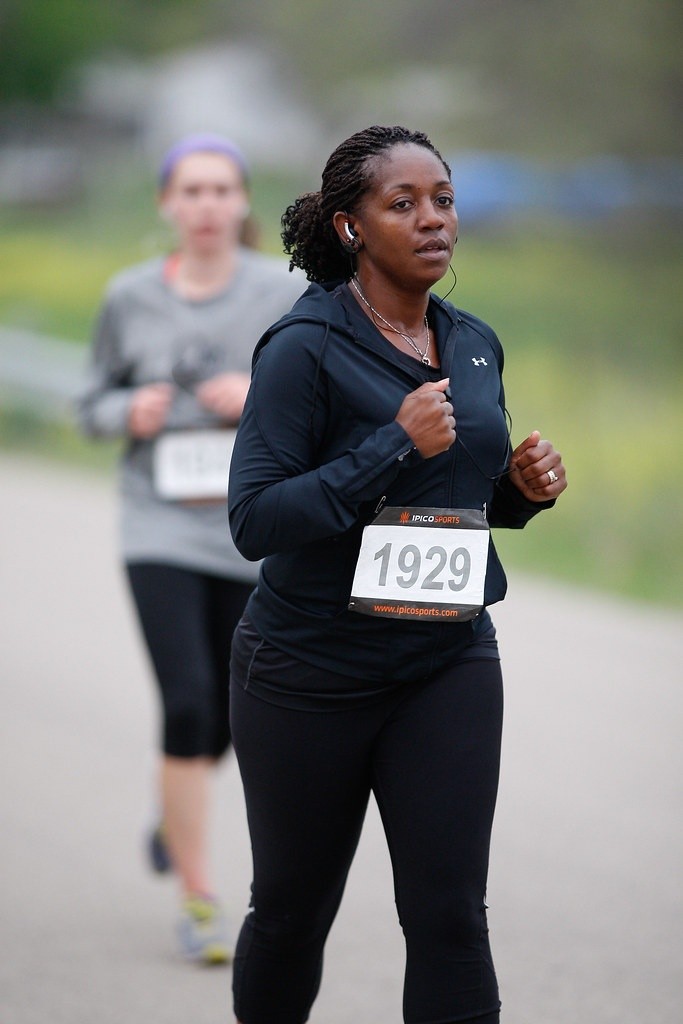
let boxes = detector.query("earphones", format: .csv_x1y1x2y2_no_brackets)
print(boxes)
343,221,358,243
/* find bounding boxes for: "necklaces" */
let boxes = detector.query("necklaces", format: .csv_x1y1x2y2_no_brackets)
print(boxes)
351,278,431,368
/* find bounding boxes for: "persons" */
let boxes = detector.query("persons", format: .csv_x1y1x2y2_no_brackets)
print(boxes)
220,129,568,1024
72,140,310,969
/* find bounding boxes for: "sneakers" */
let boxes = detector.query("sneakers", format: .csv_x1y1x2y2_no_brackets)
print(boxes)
178,903,232,965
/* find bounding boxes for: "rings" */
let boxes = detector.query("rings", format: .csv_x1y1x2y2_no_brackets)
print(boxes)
545,469,559,485
